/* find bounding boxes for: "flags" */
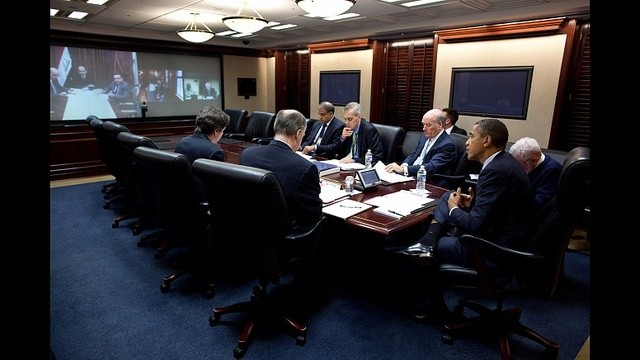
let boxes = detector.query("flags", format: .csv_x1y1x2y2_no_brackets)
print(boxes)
53,46,73,87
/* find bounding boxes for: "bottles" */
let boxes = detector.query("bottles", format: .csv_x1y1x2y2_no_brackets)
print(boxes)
415,165,427,195
364,150,374,172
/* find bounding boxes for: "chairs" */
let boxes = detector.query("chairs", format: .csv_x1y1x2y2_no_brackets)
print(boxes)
370,122,404,163
115,132,160,235
134,146,214,299
87,115,113,194
229,110,277,143
192,157,307,359
89,119,115,210
441,146,599,360
429,133,469,192
102,121,134,228
223,108,248,139
402,131,422,159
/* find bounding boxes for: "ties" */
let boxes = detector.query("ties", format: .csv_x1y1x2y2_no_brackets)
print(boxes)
417,138,432,164
115,84,118,95
341,133,357,160
314,124,327,145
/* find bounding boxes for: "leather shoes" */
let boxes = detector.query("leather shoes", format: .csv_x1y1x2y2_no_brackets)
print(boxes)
390,242,434,266
407,303,451,324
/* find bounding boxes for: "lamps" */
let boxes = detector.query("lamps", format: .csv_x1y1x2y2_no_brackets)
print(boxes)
180,3,217,42
297,0,353,20
220,0,271,37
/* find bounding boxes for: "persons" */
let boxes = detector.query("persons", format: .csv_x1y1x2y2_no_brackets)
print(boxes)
203,81,216,98
509,136,562,215
156,80,168,99
385,108,458,187
442,109,467,136
95,73,132,97
239,109,322,234
397,119,535,258
69,65,96,91
335,102,385,167
50,67,69,112
175,105,231,164
297,101,346,159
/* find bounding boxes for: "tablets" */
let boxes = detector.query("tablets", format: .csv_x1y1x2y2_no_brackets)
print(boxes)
356,167,383,189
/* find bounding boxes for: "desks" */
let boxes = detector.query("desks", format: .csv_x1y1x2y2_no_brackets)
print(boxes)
221,141,470,299
61,88,119,119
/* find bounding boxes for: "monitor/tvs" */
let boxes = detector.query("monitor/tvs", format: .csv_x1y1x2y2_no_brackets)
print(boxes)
237,76,257,97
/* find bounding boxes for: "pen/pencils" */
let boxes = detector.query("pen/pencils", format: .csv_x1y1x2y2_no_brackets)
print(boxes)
388,210,405,217
340,205,362,208
